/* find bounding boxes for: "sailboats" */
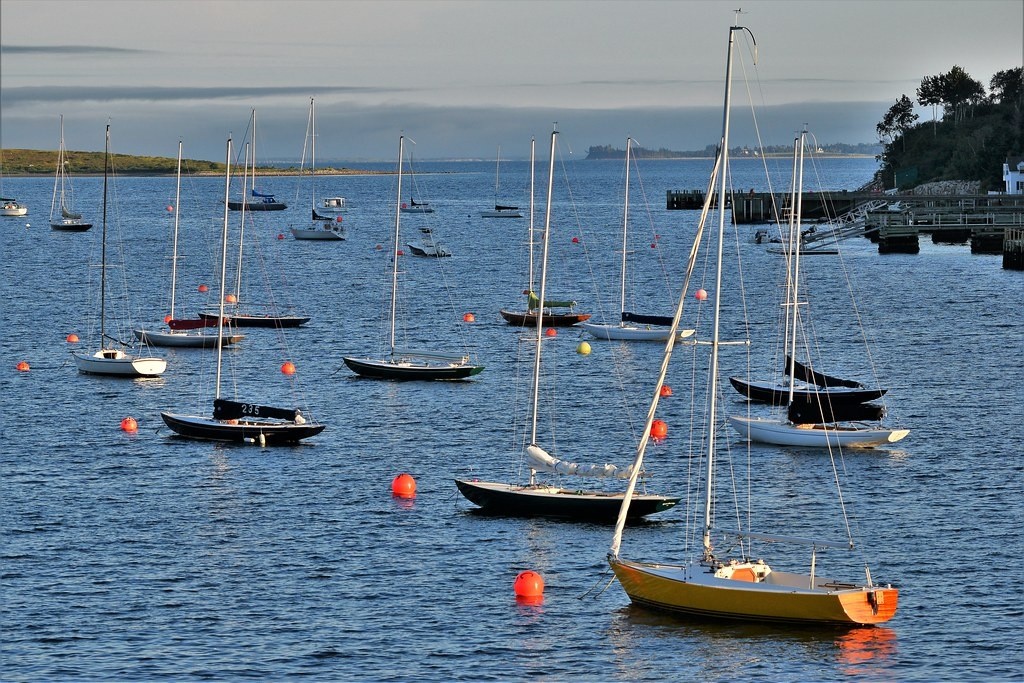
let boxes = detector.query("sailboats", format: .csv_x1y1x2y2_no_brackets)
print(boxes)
342,134,486,382
497,134,592,329
288,98,351,242
454,123,684,525
72,117,169,379
605,27,903,628
194,134,314,328
1,148,29,216
586,138,696,342
723,123,912,449
220,109,288,212
48,111,95,233
161,138,328,442
479,145,525,218
130,139,249,348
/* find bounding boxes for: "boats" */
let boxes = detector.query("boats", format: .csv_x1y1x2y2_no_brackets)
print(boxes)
748,226,772,244
